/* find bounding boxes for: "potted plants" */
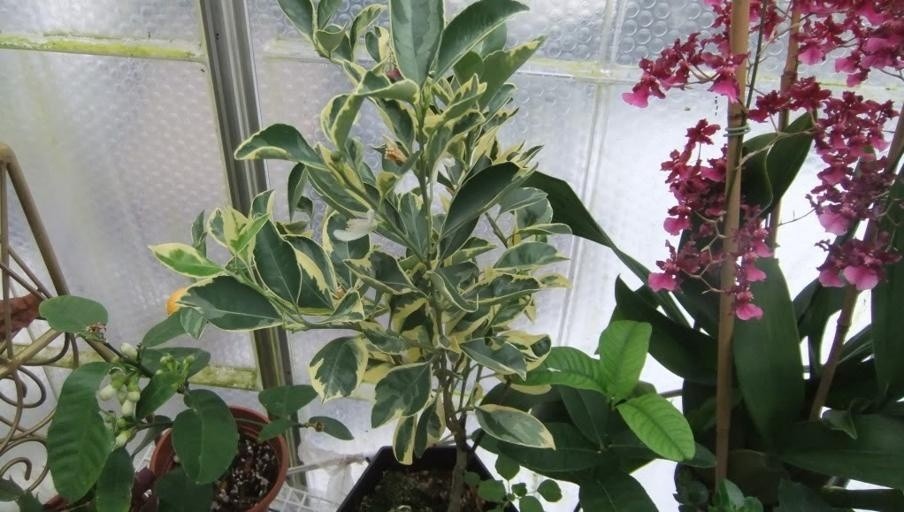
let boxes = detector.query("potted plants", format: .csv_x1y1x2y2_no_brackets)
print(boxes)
35,296,289,512
149,1,574,510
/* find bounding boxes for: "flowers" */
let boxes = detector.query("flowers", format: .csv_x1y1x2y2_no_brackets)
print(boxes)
489,0,901,512
